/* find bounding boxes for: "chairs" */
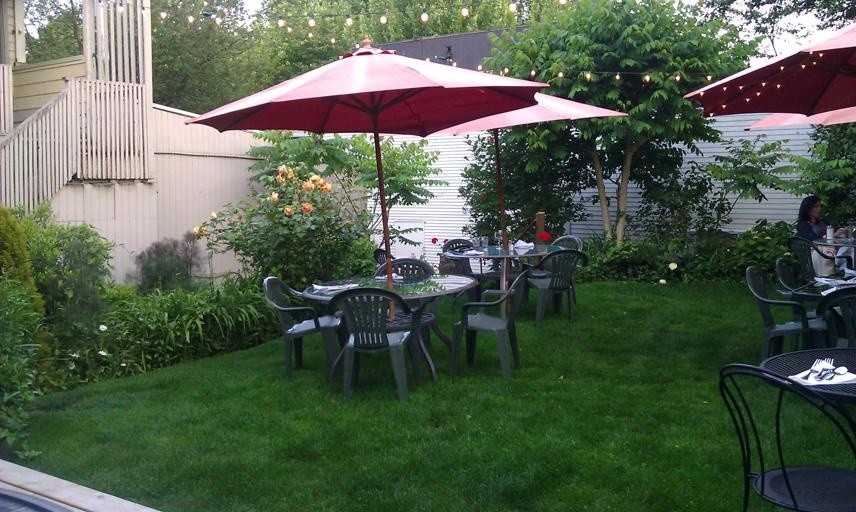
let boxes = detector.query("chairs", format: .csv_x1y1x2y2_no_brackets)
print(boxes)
787,236,836,276
374,257,436,352
327,286,439,401
551,235,583,314
374,248,395,264
522,249,588,324
448,270,529,378
262,275,340,383
717,362,855,511
442,238,486,303
745,265,826,363
775,257,814,352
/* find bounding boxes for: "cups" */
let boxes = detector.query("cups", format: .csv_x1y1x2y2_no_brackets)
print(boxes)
472,236,488,248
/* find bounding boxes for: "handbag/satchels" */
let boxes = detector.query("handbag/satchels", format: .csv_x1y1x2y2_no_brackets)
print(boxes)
811,245,838,277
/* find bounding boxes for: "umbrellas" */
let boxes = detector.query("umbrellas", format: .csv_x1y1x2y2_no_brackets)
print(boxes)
729,108,855,130
179,35,553,285
424,88,630,242
680,17,855,117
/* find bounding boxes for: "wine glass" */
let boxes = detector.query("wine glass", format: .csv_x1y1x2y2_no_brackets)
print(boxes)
495,229,504,250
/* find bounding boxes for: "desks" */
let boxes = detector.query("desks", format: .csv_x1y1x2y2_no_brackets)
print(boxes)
797,271,855,324
759,347,855,443
302,276,477,387
443,244,561,312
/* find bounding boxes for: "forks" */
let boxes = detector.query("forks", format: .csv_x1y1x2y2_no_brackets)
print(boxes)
800,357,833,379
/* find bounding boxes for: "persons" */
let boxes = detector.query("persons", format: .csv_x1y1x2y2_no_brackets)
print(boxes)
788,193,856,278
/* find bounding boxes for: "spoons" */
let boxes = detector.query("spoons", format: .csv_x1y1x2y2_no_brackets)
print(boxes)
827,365,849,380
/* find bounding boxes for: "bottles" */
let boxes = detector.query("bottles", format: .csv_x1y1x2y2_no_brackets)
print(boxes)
826,224,835,239
